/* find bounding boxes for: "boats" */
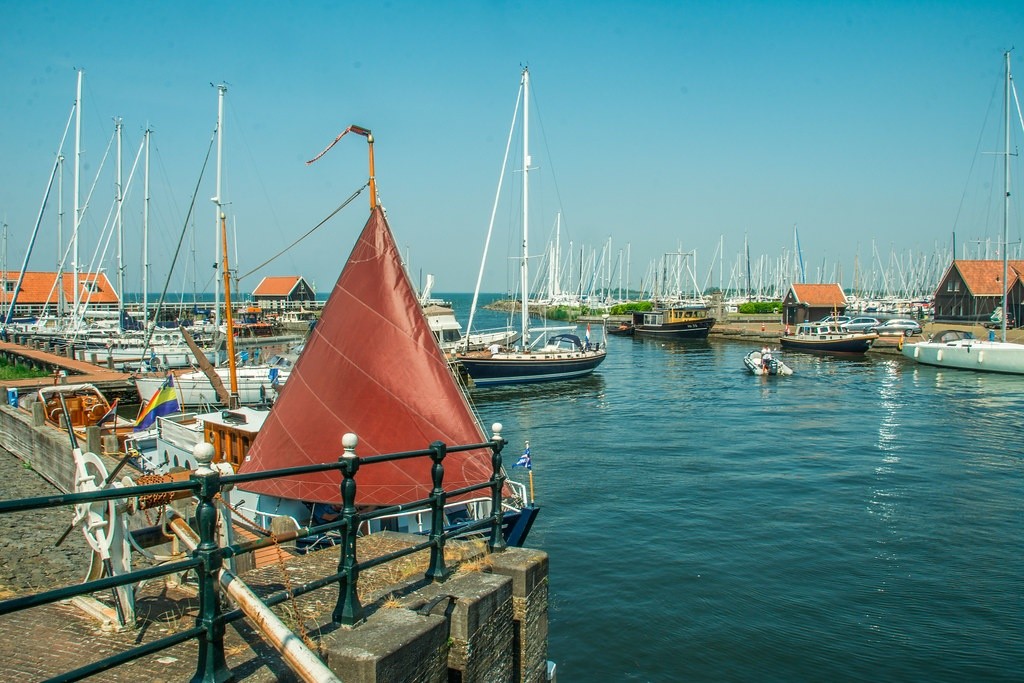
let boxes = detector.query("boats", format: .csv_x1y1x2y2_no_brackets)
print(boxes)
608,321,635,337
782,321,878,357
631,306,717,342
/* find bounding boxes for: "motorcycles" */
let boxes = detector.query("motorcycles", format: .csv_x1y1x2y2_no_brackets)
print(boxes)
743,346,794,376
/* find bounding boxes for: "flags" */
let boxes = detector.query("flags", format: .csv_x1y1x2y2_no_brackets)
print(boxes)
585,322,590,351
512,448,532,470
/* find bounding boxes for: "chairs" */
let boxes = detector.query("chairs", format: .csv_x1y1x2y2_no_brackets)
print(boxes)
87,404,106,427
49,407,65,422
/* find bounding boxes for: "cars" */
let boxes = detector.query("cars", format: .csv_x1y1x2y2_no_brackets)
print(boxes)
864,318,922,337
837,317,880,334
818,316,851,325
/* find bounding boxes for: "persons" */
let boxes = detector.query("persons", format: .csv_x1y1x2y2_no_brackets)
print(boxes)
761,344,772,362
484,341,504,357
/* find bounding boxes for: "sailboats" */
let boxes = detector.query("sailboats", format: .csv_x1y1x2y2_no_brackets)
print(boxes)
609,226,901,316
0,53,608,552
901,53,1024,379
